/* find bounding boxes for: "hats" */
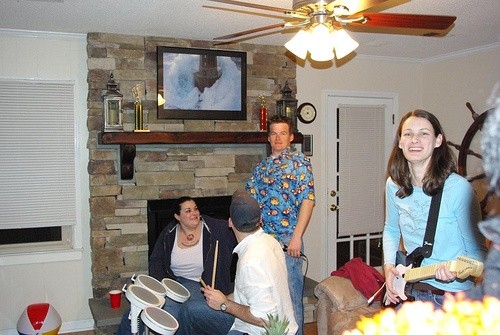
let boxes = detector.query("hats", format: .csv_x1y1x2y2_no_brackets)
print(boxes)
229,188,262,233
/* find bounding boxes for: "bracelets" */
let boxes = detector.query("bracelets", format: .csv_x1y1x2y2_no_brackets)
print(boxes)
181,234,199,247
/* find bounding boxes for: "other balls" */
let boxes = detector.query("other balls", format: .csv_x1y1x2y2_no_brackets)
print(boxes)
17,302,62,335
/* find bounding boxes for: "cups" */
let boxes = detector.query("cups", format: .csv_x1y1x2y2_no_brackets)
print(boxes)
108,290,122,309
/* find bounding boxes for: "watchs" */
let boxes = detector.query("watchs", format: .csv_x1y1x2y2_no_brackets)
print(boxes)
221,299,231,312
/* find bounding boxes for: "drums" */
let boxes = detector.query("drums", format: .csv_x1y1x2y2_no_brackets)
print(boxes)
125,284,162,309
161,278,190,303
140,306,179,335
134,274,167,298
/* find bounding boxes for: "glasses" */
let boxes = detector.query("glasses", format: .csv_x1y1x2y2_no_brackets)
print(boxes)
267,131,289,137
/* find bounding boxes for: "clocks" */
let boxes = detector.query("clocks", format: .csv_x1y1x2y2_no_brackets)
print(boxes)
298,103,318,124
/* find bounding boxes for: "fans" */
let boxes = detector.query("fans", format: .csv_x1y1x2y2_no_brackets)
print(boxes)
203,0,457,40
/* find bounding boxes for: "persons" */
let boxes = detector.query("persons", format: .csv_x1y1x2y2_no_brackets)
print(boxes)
116,196,238,335
383,110,489,310
177,191,300,335
245,115,314,335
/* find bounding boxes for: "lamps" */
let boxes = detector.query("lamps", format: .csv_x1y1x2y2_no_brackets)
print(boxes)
276,81,298,131
282,24,359,63
100,72,124,133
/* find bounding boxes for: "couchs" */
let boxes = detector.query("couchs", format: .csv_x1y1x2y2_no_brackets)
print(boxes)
312,262,386,335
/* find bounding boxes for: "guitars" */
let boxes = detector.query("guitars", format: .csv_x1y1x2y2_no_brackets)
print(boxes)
380,250,485,307
